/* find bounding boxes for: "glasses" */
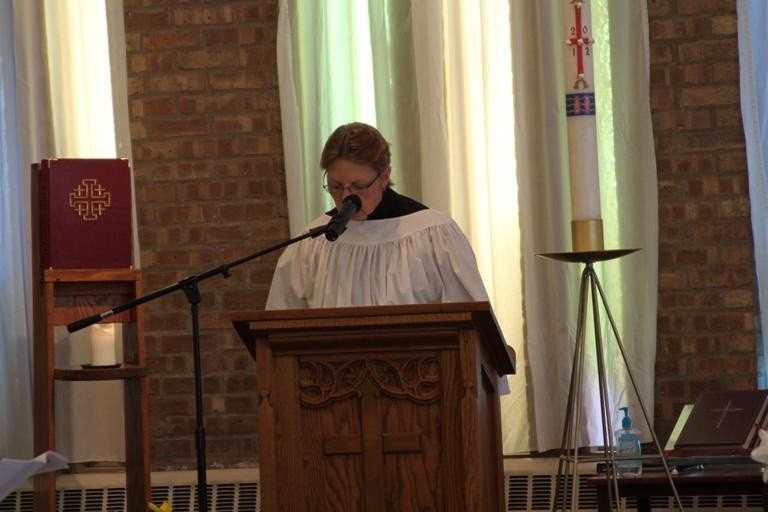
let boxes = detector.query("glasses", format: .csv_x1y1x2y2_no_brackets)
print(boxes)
322,169,383,193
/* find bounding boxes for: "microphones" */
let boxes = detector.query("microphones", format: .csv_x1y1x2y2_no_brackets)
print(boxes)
325,194,362,242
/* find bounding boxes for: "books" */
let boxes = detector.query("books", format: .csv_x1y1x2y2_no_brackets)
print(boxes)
0,449,71,502
659,385,767,460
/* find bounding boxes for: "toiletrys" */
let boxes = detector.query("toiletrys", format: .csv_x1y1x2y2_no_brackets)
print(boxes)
615,406,642,475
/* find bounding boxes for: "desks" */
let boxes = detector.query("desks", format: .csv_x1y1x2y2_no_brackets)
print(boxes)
586,466,767,510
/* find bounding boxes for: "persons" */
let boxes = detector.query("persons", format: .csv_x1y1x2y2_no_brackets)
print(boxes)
264,121,512,399
